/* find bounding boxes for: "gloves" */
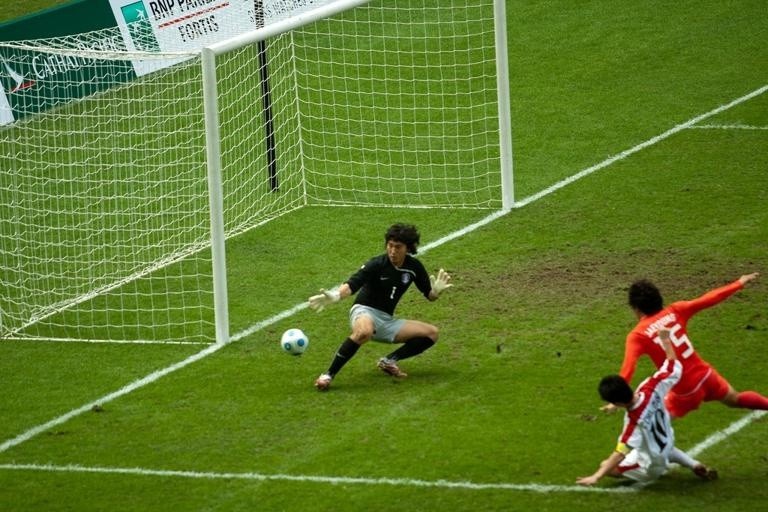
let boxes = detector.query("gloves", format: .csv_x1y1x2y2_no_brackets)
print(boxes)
430,269,453,297
308,288,340,313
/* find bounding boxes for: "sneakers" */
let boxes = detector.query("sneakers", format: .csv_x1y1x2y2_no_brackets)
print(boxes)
692,464,717,481
316,373,333,391
377,357,407,377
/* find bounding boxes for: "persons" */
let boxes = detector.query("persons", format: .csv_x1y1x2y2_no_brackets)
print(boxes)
306,222,455,390
599,271,768,415
573,327,718,486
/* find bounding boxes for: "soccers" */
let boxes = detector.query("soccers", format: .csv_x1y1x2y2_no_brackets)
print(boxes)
282,329,308,355
